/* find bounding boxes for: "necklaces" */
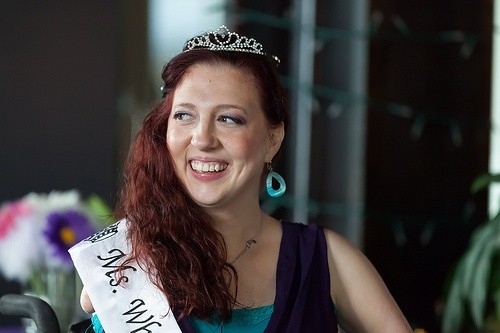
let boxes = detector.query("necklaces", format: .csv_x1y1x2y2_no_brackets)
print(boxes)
229,215,264,266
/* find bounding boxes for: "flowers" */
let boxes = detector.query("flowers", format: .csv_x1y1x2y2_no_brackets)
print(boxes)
0,189,116,295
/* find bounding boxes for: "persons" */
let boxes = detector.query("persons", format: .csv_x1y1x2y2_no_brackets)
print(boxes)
79,24,414,333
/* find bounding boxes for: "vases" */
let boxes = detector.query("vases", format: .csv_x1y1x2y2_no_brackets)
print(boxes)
31,270,91,333
